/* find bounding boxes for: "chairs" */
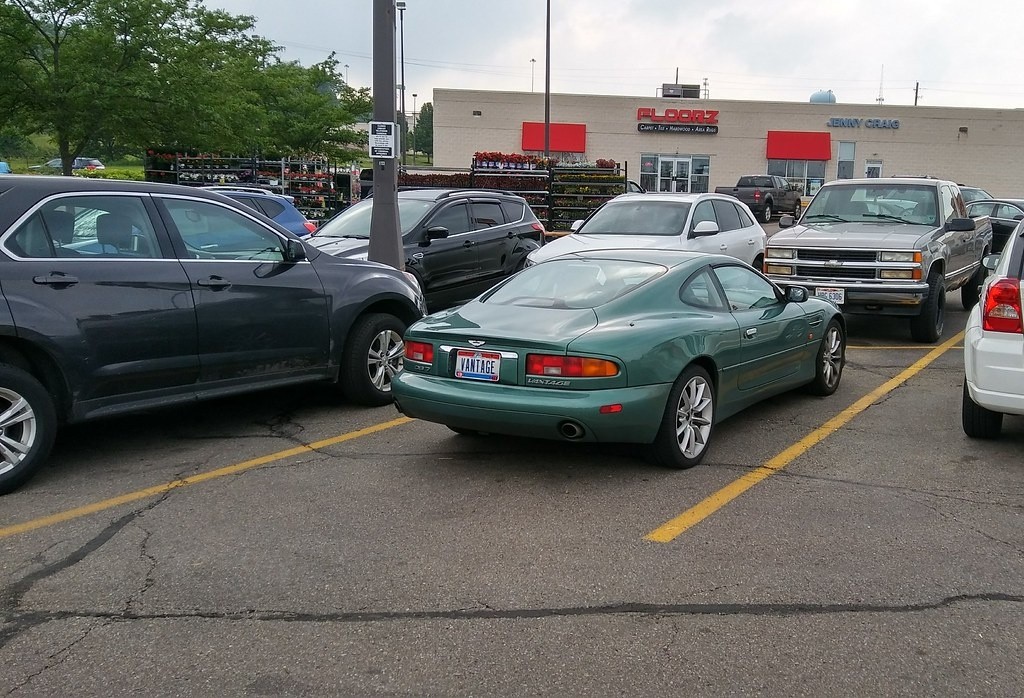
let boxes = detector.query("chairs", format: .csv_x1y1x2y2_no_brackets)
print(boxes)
913,202,936,217
847,201,869,215
96,214,138,257
44,210,81,257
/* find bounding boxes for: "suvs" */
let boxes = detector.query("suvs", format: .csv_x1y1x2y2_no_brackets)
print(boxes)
299,186,547,316
52,185,317,256
28,156,105,172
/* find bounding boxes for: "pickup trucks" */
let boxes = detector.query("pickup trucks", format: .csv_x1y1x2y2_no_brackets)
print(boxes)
763,175,994,344
715,174,802,223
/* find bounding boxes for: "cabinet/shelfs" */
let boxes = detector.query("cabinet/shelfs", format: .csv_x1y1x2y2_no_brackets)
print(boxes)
470,158,549,221
551,161,628,232
281,156,330,222
144,152,176,183
176,152,259,188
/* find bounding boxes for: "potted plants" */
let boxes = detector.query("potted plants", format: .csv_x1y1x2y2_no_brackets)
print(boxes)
268,176,278,186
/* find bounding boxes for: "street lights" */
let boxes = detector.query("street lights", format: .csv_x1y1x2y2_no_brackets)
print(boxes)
395,85,406,113
412,94,417,164
530,58,536,92
344,64,350,130
396,2,407,174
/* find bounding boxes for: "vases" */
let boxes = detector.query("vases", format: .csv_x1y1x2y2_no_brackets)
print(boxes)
302,189,316,195
477,161,536,169
286,177,325,181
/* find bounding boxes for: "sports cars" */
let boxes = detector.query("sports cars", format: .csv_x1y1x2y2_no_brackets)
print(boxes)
390,250,847,470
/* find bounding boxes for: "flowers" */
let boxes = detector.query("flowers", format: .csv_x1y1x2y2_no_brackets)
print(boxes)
554,159,625,230
147,149,182,182
284,172,333,179
294,186,323,192
473,151,541,164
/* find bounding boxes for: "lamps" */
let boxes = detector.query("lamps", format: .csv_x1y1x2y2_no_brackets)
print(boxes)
959,127,968,132
473,111,481,115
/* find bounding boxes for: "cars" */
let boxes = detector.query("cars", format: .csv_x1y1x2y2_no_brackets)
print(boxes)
521,191,767,287
0,174,429,495
0,162,12,174
961,217,1024,441
865,185,995,219
964,199,1024,253
